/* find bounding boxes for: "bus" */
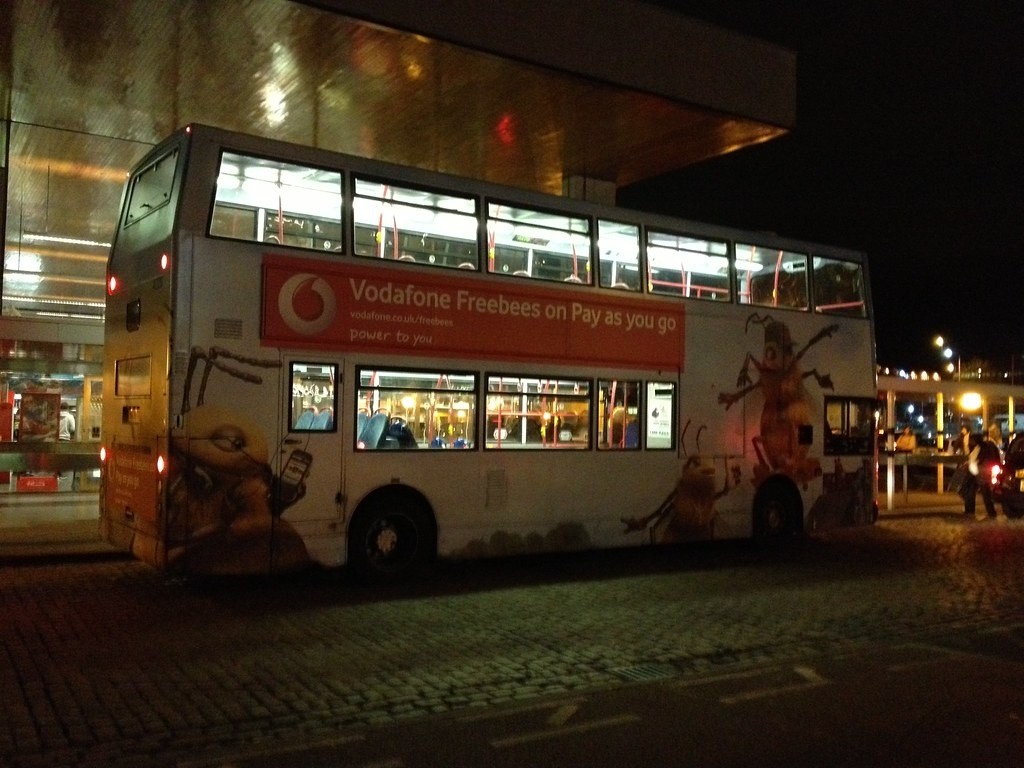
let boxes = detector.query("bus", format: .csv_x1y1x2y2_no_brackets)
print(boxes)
97,122,879,584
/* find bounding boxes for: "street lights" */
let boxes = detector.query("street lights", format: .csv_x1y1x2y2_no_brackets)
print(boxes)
935,336,944,382
959,392,989,443
944,348,961,382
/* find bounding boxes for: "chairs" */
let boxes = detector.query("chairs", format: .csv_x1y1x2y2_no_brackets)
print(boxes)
295,406,471,449
264,235,631,289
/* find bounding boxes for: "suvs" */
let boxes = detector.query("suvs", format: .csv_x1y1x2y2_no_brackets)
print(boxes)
994,434,1024,518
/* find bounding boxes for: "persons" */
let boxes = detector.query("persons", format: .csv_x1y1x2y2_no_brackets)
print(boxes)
954,423,1001,519
14,408,20,438
896,424,916,451
56,403,76,479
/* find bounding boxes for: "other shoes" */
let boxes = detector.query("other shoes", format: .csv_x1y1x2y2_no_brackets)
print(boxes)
988,511,997,518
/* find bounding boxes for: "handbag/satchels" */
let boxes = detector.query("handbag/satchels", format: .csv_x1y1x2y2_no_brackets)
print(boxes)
948,468,974,499
